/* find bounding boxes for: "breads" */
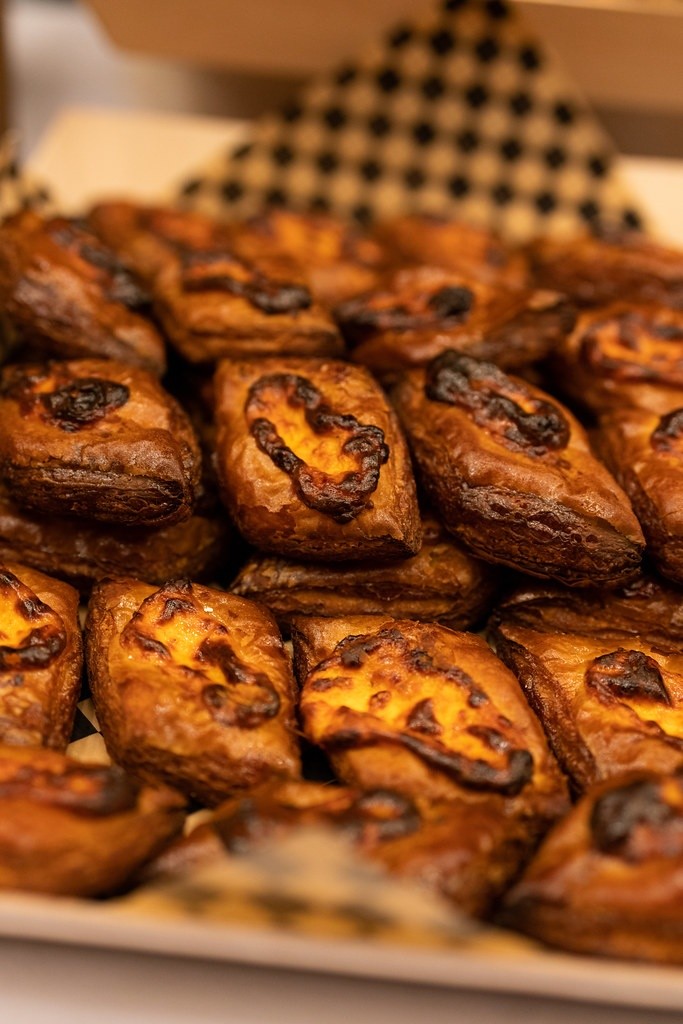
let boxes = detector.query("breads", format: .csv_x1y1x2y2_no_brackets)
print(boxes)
0,203,683,966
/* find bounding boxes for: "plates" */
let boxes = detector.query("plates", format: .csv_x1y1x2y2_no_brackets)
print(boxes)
0,101,683,1010
